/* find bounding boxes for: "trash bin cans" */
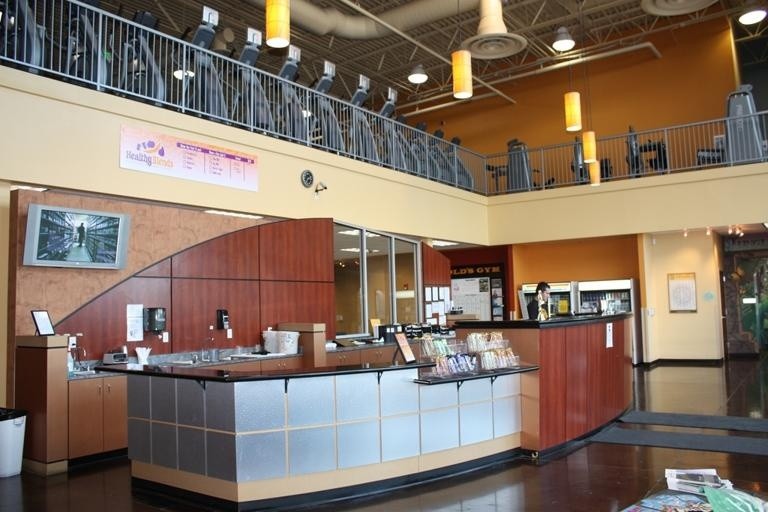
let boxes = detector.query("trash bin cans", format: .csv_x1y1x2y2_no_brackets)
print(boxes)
0,407,30,479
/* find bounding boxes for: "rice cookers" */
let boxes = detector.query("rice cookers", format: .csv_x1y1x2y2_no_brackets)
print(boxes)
263,329,300,354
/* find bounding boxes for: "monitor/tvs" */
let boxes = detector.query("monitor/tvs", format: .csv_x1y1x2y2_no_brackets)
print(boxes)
22,203,132,270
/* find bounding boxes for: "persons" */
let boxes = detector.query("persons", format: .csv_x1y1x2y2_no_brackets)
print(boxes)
77,222,86,246
527,281,554,319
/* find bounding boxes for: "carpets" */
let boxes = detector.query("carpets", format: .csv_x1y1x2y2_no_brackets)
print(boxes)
619,410,768,434
592,425,768,458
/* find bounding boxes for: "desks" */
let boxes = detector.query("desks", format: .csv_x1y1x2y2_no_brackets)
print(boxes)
643,470,768,512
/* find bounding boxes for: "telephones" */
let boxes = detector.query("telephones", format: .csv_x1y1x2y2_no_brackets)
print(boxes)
216,309,229,330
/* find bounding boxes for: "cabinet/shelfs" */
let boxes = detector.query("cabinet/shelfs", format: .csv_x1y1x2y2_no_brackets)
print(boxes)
37,211,74,260
326,348,361,367
395,341,421,362
360,344,399,363
261,356,305,372
68,375,129,480
201,361,261,373
86,215,119,263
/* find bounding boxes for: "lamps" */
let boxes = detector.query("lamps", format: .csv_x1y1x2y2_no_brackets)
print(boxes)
683,223,745,238
409,63,430,85
450,0,472,100
265,0,291,48
738,0,768,26
577,1,597,163
563,1,583,133
550,25,576,52
579,1,602,187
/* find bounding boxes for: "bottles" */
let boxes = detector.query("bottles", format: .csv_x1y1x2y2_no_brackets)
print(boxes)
209,349,219,362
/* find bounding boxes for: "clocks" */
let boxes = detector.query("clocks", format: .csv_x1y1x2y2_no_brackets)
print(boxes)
301,170,314,187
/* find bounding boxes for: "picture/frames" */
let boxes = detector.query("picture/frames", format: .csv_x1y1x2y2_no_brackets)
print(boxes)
31,310,56,336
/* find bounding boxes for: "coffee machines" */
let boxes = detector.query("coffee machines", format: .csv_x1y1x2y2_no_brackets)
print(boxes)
62,333,94,375
